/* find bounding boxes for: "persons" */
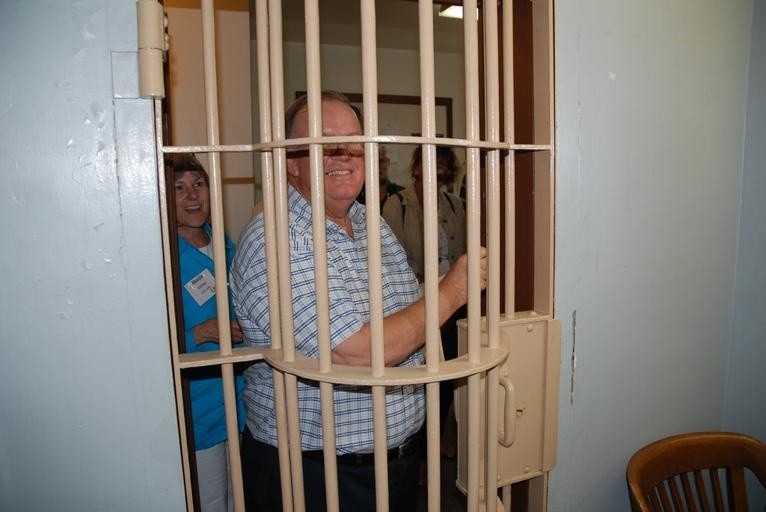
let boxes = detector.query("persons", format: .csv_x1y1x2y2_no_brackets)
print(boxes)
230,88,489,511
382,140,467,485
356,148,407,214
169,151,250,512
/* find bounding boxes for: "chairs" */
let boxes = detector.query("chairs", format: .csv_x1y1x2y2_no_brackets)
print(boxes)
627,431,766,511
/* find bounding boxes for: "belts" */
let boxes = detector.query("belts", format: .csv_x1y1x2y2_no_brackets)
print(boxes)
241,422,422,461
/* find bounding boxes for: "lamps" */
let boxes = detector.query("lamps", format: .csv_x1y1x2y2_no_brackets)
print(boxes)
439,2,479,21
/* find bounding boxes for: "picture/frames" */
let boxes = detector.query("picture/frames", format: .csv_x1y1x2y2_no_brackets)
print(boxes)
295,90,453,195
